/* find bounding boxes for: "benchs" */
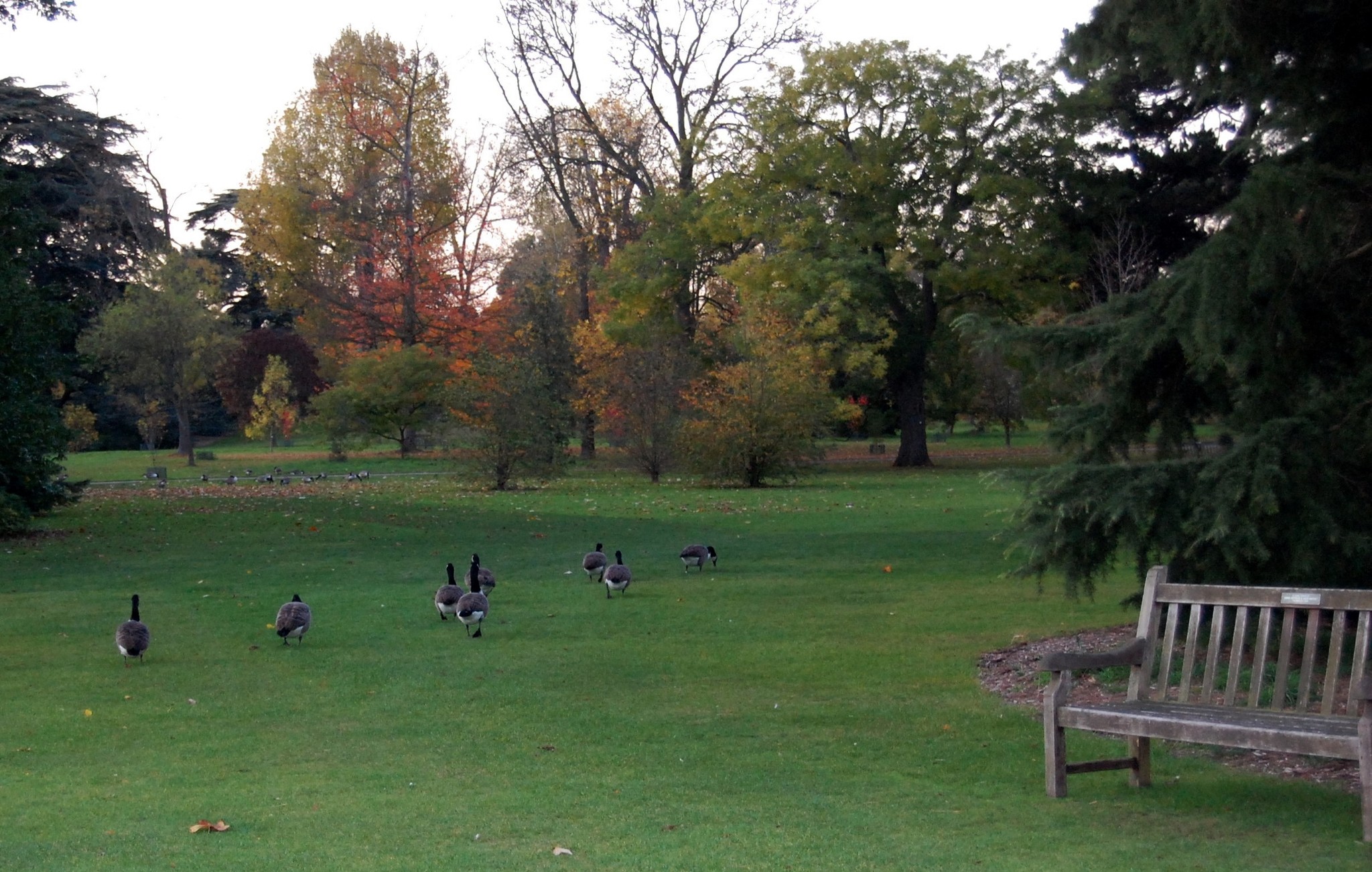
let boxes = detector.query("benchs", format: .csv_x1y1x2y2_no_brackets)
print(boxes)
1041,564,1372,848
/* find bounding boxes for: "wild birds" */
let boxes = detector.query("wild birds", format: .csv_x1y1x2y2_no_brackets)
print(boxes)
302,477,314,483
158,479,167,489
316,473,327,480
434,563,463,620
254,474,274,484
288,470,305,476
141,473,159,479
117,594,149,667
276,595,311,645
680,545,717,573
604,551,631,599
464,555,495,597
582,544,606,583
201,475,208,481
223,475,238,485
342,471,369,481
245,470,253,475
273,466,283,475
455,565,489,637
280,478,291,485
